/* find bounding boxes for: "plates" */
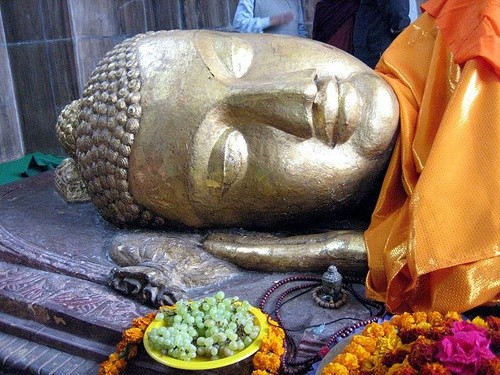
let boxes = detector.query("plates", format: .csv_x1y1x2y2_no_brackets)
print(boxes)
142,304,268,371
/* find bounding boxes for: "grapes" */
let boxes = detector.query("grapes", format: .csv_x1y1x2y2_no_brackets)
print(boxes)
148,291,261,360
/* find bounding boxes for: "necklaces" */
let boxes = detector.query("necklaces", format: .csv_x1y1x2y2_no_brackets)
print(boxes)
260,274,390,375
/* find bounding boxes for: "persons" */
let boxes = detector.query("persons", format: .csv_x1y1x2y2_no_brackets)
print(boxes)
54,0,500,319
233,0,308,39
322,264,343,299
312,0,413,72
